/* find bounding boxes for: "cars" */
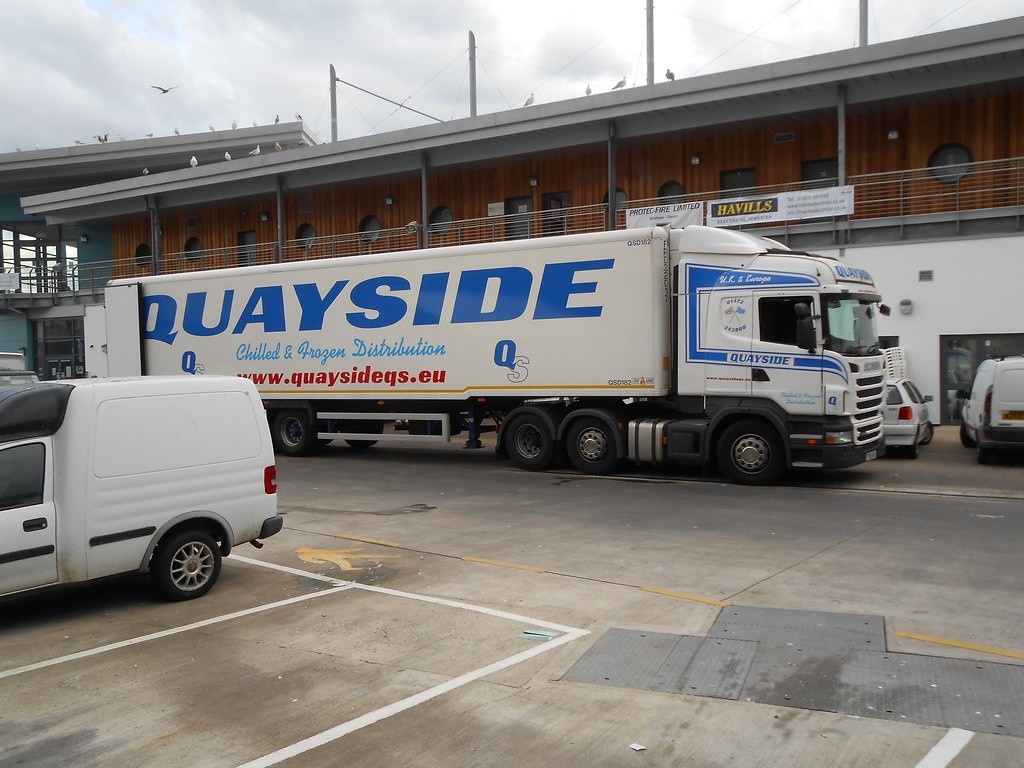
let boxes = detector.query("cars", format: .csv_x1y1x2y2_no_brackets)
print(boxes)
882,378,934,460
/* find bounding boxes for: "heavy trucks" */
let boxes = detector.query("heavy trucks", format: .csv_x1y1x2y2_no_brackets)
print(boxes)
82,225,892,486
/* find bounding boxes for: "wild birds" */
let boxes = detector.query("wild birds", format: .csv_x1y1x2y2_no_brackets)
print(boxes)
522,68,675,107
15,85,311,175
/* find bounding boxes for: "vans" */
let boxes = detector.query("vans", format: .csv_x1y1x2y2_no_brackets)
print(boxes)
0,371,40,389
953,353,1024,466
948,345,973,423
0,375,285,605
